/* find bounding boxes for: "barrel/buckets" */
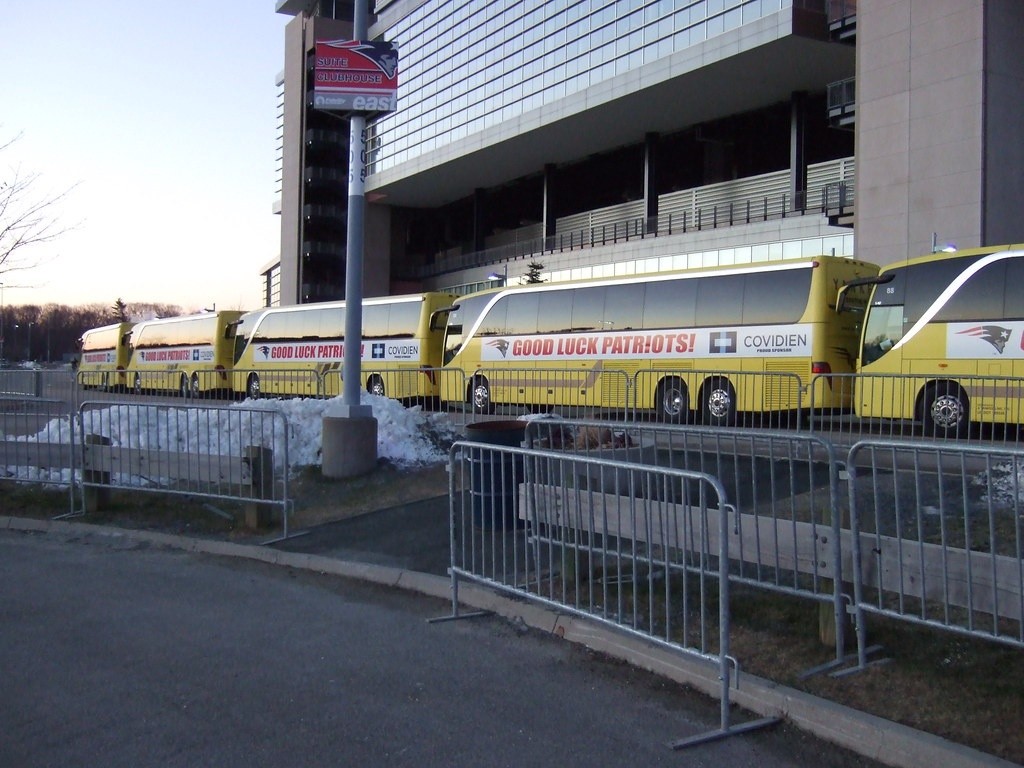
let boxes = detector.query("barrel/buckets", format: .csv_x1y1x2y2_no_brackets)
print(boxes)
466,420,533,532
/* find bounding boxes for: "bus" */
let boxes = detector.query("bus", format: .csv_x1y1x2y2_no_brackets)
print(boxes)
73,323,139,392
835,244,1023,439
120,310,253,399
223,292,460,401
427,254,884,425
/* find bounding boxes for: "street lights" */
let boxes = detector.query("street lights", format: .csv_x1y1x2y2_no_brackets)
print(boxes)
28,321,38,361
